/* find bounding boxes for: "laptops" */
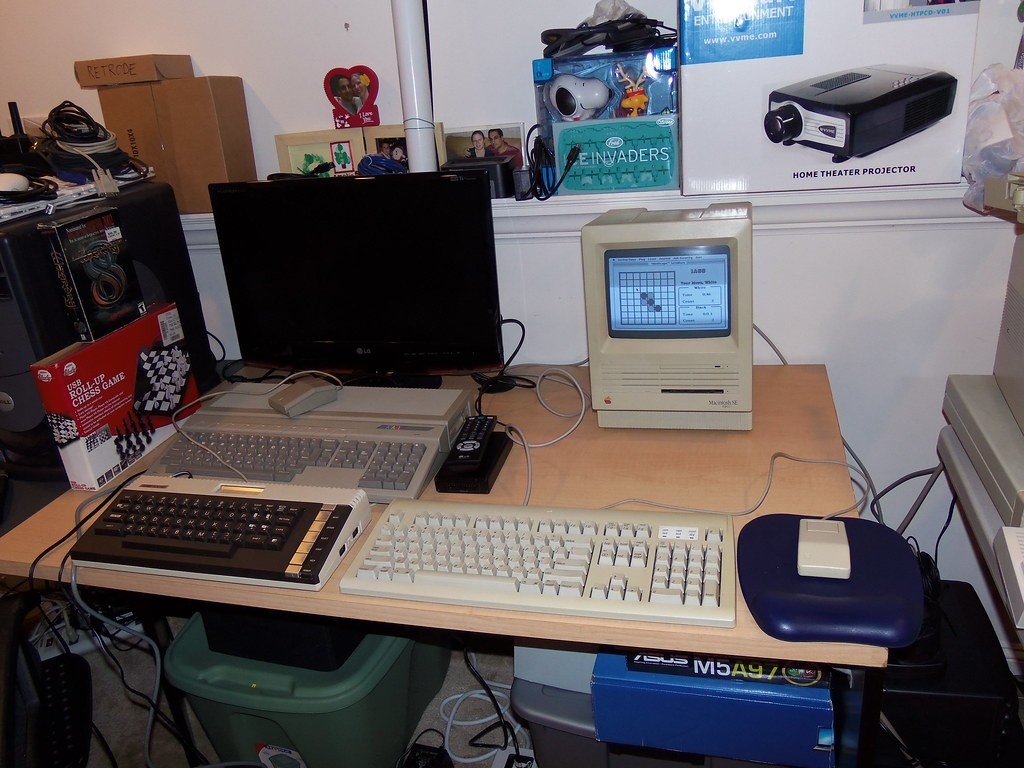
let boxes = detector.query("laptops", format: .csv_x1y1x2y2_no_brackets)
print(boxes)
0,167,156,224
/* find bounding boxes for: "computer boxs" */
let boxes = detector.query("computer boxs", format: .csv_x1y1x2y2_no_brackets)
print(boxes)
0,180,218,481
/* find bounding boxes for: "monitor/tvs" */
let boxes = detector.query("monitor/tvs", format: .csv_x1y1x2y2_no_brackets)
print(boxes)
580,207,754,431
206,170,506,389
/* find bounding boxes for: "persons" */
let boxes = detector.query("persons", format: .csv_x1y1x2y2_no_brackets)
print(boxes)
464,130,494,158
381,139,392,158
486,128,523,165
333,73,369,116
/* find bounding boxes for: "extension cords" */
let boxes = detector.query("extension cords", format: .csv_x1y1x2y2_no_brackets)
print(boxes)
491,745,537,768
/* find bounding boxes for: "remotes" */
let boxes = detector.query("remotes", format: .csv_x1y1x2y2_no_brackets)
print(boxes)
446,415,497,469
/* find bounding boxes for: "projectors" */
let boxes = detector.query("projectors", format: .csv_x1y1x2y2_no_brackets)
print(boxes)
764,64,958,162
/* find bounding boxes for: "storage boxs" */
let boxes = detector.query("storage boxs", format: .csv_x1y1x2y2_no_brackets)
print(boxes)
32,301,202,492
73,54,195,88
533,47,677,194
676,0,983,197
98,76,257,214
511,645,836,768
165,614,449,768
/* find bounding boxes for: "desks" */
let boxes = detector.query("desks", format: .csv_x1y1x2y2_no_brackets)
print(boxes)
0,363,891,768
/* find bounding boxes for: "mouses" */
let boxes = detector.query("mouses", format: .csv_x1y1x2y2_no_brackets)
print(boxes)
798,518,851,580
267,377,338,418
0,173,29,192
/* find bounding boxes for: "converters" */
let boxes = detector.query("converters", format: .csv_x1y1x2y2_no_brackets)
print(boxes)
403,744,454,768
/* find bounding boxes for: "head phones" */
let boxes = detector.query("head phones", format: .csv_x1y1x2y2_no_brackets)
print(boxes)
541,15,664,57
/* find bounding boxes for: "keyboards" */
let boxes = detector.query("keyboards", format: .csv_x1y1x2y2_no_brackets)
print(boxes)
145,411,454,504
69,476,374,591
339,500,736,627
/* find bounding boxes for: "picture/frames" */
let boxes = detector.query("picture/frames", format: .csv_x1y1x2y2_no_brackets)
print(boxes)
365,122,447,173
324,66,380,129
444,121,526,172
274,127,365,178
